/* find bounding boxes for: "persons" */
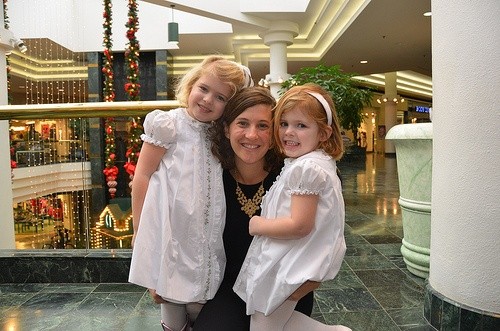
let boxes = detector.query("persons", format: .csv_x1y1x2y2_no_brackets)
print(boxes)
233,82,353,331
128,54,251,331
147,86,320,331
51,229,64,249
64,228,69,244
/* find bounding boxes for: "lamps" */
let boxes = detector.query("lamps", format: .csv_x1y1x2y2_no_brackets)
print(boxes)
167,5,178,41
376,96,405,105
